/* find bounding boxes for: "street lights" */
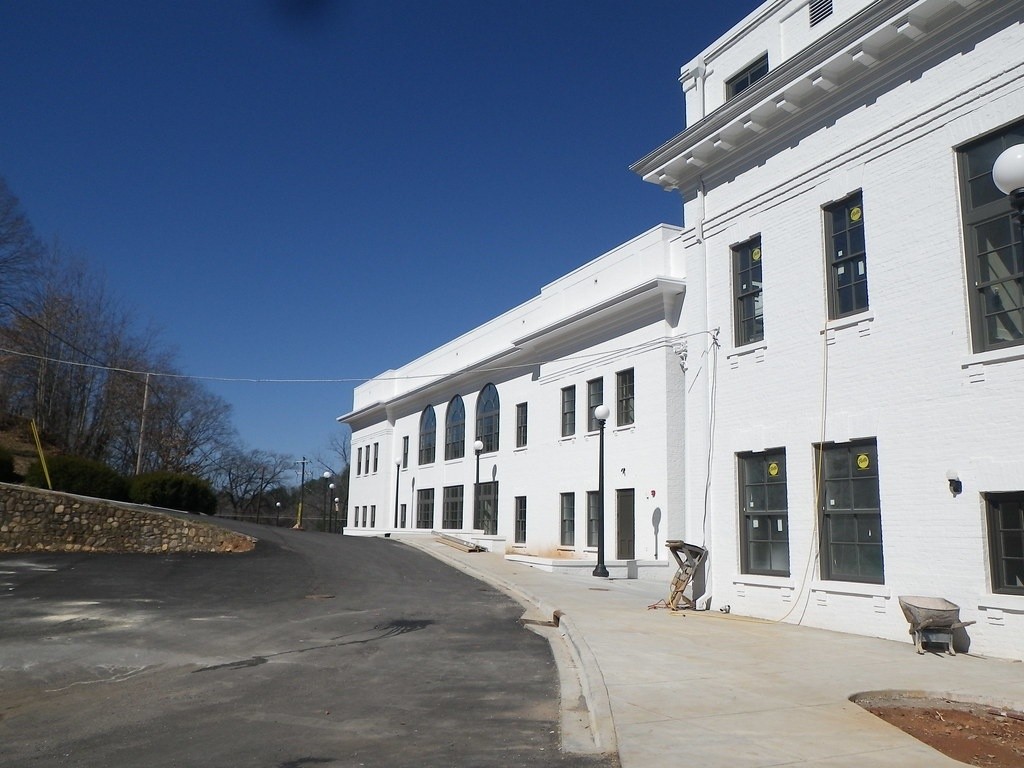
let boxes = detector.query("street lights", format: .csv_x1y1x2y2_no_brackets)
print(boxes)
335,497,339,533
323,472,332,532
473,440,484,529
394,457,403,528
328,483,335,533
593,405,610,577
275,501,281,527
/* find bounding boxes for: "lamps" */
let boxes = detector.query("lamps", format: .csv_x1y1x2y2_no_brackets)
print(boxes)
946,470,962,493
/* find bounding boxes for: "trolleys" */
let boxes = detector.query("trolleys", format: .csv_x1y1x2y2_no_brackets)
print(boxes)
898,595,977,656
